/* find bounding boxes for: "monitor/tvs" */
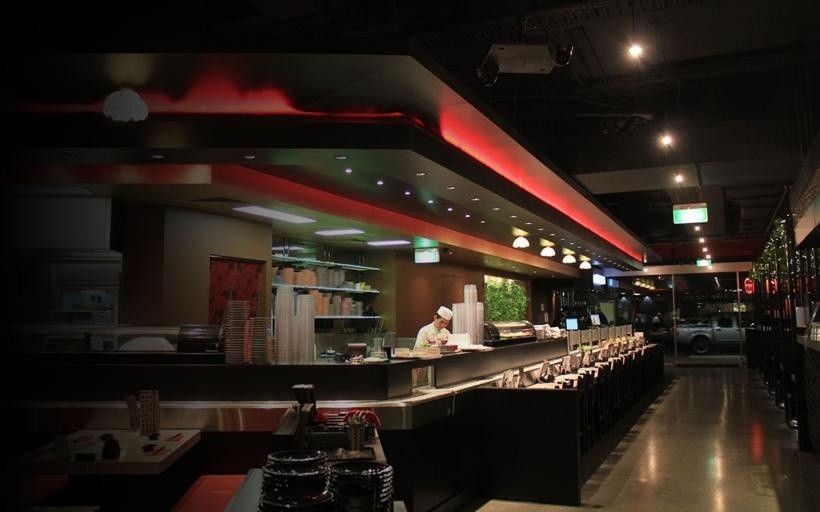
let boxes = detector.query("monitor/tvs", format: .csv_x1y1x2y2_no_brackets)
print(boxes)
590,314,601,326
565,316,579,330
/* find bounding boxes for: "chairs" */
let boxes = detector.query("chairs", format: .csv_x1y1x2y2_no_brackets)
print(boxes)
555,342,665,455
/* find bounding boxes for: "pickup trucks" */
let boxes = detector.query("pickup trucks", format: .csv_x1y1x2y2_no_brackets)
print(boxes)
672,312,771,355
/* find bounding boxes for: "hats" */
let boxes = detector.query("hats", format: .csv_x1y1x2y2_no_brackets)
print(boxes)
437,306,453,320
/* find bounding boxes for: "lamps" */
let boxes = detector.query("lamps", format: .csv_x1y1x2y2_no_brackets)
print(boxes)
579,261,592,270
540,246,556,257
512,235,530,249
562,254,576,264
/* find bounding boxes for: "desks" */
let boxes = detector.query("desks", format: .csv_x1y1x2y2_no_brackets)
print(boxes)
169,473,265,512
9,430,201,475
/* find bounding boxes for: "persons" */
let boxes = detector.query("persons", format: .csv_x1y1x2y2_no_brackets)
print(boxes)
413,306,454,350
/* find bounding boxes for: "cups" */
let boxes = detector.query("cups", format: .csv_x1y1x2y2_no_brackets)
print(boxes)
275,265,378,316
219,283,316,365
533,322,567,341
451,283,486,348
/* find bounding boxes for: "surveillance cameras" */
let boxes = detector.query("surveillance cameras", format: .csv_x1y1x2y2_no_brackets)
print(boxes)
548,37,574,66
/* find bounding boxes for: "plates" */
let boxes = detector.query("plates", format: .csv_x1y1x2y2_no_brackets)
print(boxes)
319,336,462,365
254,448,396,510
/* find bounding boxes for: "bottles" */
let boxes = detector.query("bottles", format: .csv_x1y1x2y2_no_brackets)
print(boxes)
99,438,121,461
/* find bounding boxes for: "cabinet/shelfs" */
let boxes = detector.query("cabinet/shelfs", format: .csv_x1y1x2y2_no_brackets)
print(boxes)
272,255,382,319
